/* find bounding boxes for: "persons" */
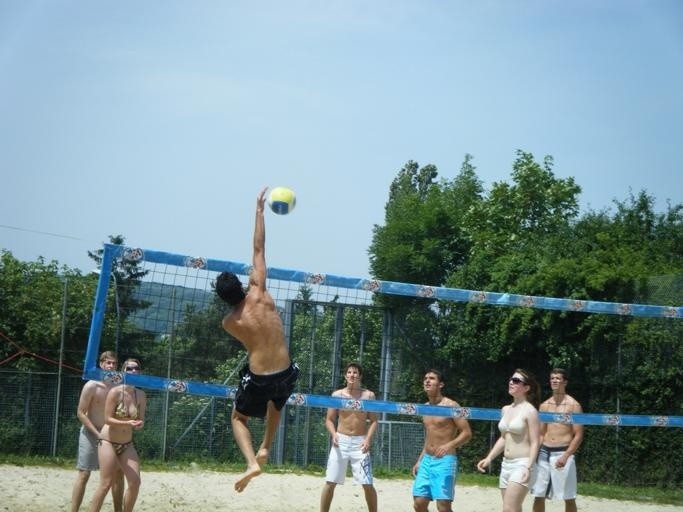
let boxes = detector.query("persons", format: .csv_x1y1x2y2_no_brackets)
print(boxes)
531,367,583,511
69,350,123,512
84,358,147,511
316,362,379,511
476,366,545,511
410,365,473,511
212,184,297,492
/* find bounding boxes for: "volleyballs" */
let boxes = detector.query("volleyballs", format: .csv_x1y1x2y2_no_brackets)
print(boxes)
268,185,296,214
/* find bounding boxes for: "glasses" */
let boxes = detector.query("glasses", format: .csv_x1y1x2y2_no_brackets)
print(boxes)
509,377,526,385
126,365,140,371
